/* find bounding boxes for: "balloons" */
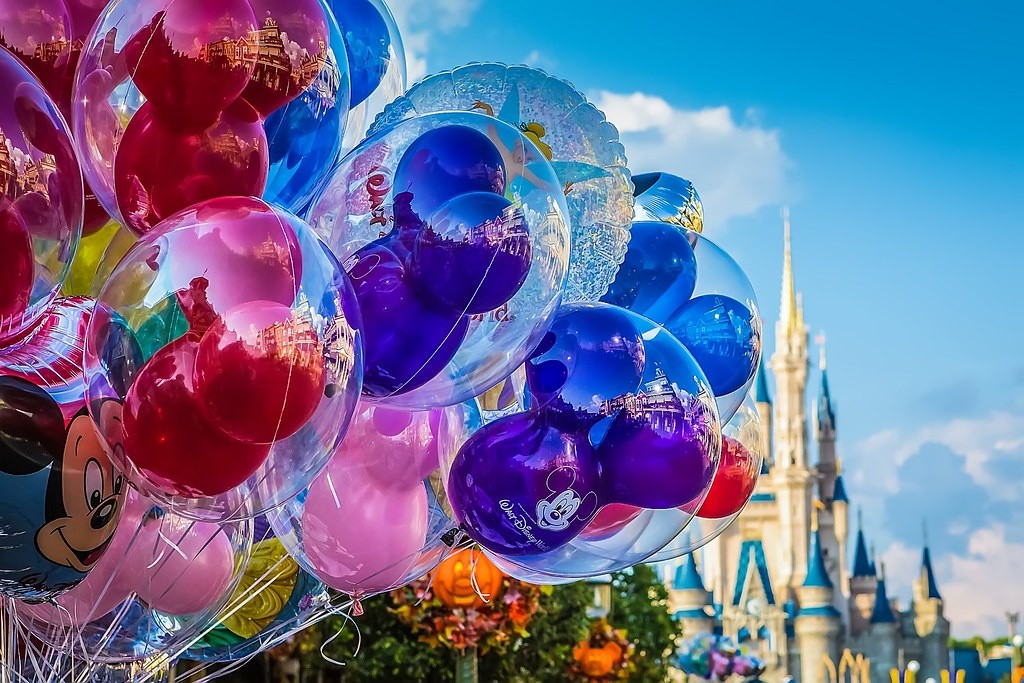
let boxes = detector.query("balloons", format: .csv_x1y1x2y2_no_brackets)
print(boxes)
0,0,765,683
